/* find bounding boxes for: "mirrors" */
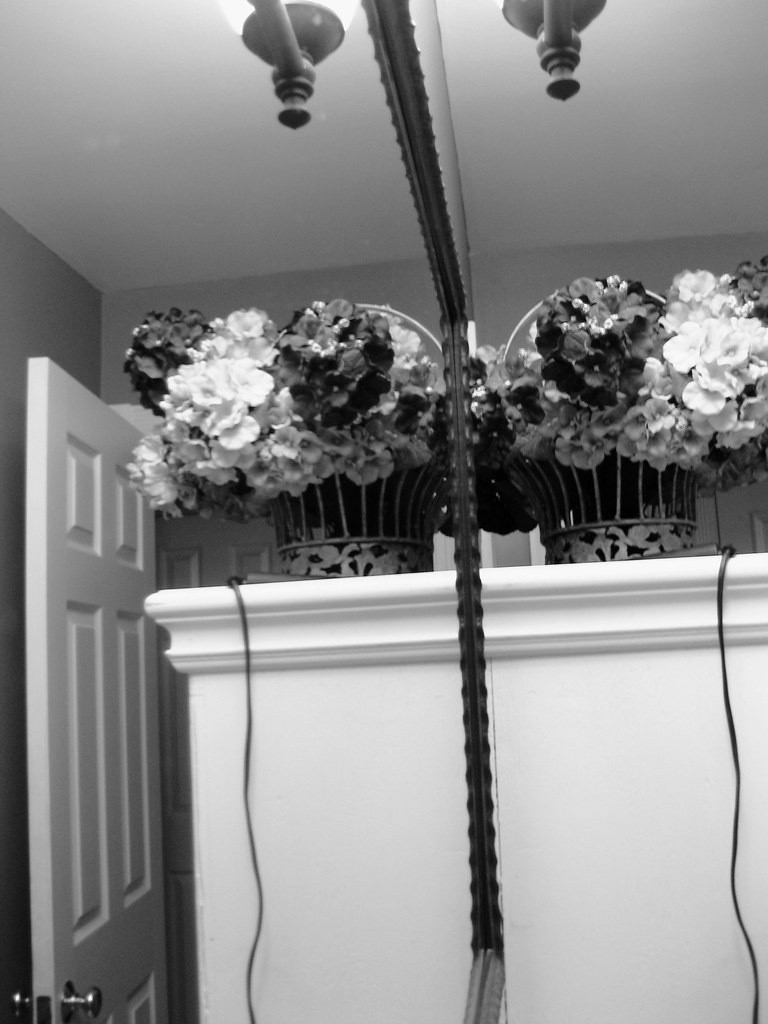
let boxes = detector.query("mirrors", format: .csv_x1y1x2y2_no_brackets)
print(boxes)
1,0,507,1024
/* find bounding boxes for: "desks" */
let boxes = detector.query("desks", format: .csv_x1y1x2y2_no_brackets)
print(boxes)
143,570,474,1024
478,551,768,1023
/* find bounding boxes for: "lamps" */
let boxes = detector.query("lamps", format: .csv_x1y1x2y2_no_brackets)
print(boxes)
498,0,607,101
216,1,361,132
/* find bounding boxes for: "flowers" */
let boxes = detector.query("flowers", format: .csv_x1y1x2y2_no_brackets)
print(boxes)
124,307,451,525
468,255,768,536
